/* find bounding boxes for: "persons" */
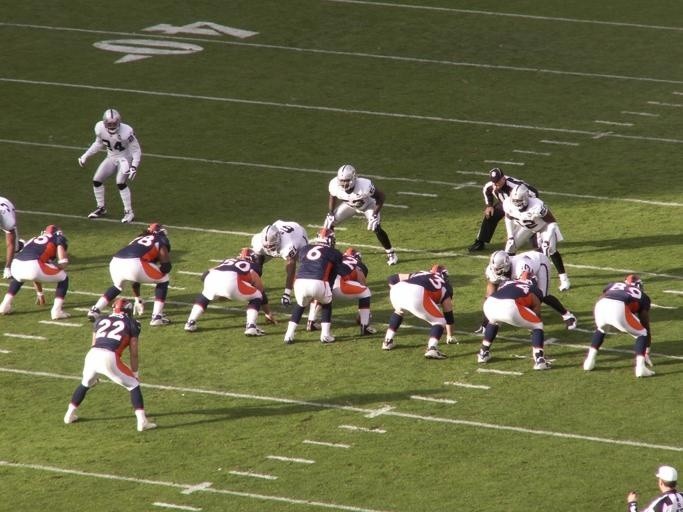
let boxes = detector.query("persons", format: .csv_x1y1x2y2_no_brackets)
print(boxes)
182,247,278,337
0,196,19,278
86,222,172,327
581,274,657,377
304,245,377,338
61,297,156,433
379,263,460,359
319,163,399,267
250,219,309,309
502,183,571,293
76,108,141,223
280,228,343,344
0,224,72,320
476,271,554,371
473,249,578,336
464,166,539,252
627,463,682,512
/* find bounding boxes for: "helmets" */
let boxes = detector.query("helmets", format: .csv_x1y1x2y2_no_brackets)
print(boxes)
111,298,133,318
260,225,281,257
337,165,357,192
41,224,64,237
235,247,260,264
315,228,335,249
624,274,644,291
519,271,537,287
489,250,512,277
146,222,168,236
102,109,121,135
343,248,362,261
510,184,529,210
431,265,448,279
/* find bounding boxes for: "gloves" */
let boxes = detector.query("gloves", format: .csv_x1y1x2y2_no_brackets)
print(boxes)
35,295,44,305
122,167,137,183
367,214,380,231
446,335,458,344
324,213,335,229
2,267,12,279
280,293,291,306
541,240,550,256
507,239,516,250
133,296,144,316
78,156,86,168
476,320,488,334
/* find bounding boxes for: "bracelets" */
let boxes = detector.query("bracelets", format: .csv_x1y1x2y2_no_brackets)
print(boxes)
35,290,44,296
131,370,138,378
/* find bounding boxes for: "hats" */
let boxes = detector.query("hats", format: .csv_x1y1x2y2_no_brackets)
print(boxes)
490,167,503,183
655,465,678,481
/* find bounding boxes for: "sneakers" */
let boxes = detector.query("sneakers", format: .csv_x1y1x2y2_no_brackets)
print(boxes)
533,357,552,370
87,206,107,218
467,241,484,253
381,339,397,350
0,303,14,315
558,277,571,291
564,314,577,330
87,305,100,321
244,323,266,336
360,327,378,336
283,333,297,343
184,319,198,332
121,210,135,223
137,423,157,431
635,367,656,378
385,250,398,265
476,350,492,363
583,359,595,371
51,310,72,320
306,319,321,331
64,415,79,424
423,346,449,359
150,313,170,326
320,336,335,342
